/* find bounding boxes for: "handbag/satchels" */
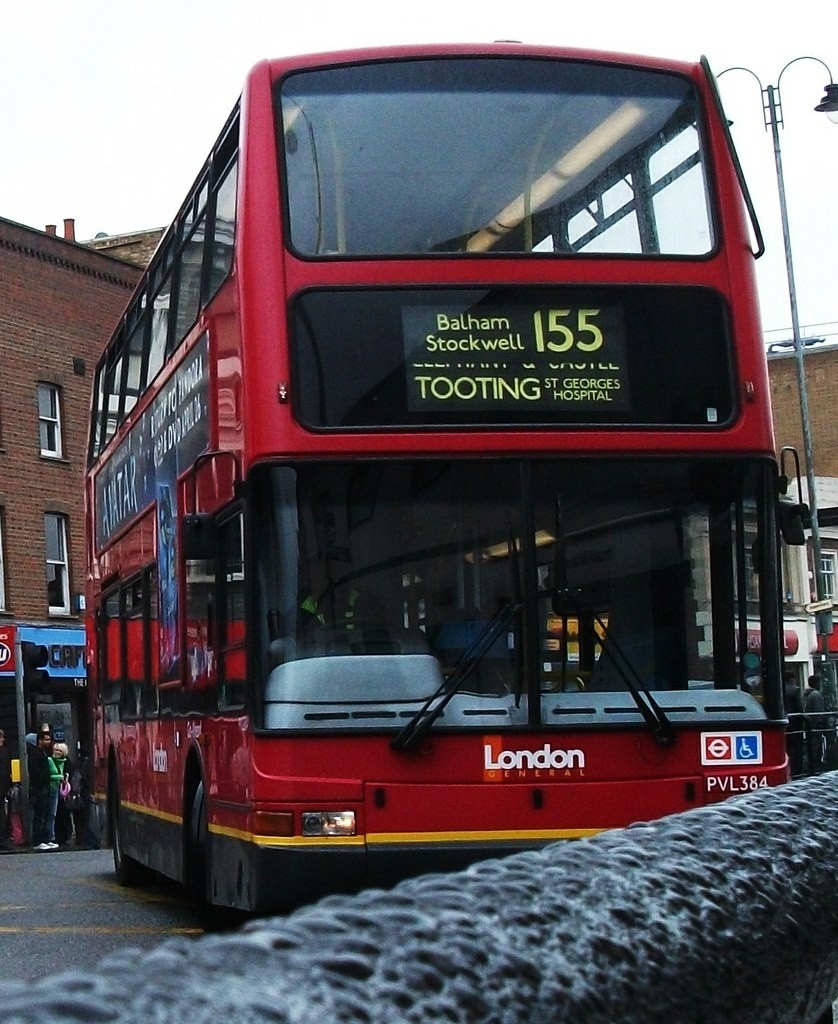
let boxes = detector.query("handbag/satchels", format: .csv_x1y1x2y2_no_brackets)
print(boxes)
60,781,71,796
62,769,86,809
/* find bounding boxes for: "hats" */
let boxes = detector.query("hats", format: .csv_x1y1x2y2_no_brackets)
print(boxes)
54,743,68,757
24,733,36,745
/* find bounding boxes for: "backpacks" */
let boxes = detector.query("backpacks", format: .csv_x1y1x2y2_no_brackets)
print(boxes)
9,785,22,814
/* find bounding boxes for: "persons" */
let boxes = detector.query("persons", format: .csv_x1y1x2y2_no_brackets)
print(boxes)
804,675,827,772
51,743,73,844
0,730,13,851
298,581,362,630
27,733,65,851
784,672,807,773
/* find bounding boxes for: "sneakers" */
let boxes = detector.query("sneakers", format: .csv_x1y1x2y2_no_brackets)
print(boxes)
33,843,51,849
46,841,59,848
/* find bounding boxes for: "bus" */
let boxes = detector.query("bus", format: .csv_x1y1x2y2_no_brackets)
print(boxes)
85,40,809,913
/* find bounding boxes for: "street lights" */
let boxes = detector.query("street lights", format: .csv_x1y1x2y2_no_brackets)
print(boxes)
717,56,838,711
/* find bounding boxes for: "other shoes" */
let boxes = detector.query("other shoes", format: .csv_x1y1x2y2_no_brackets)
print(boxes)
0,837,22,851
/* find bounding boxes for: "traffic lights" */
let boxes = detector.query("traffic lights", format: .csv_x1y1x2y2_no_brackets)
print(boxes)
21,640,51,702
